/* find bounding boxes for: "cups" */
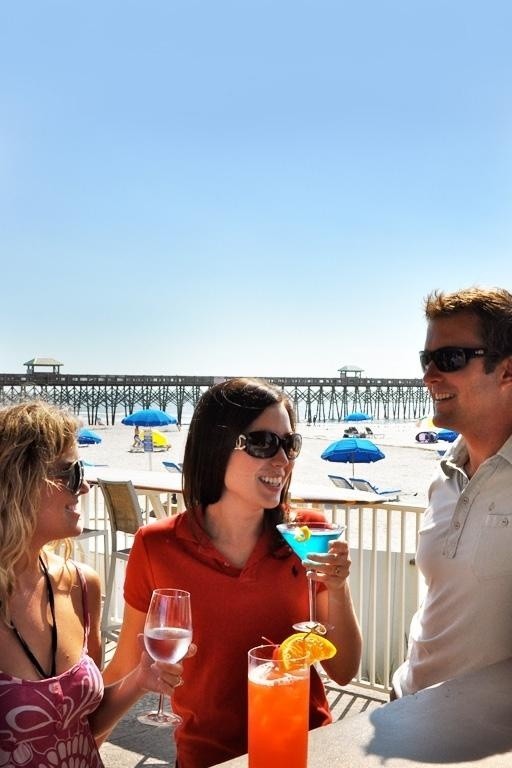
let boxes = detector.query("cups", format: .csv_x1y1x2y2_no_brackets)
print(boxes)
248,644,311,768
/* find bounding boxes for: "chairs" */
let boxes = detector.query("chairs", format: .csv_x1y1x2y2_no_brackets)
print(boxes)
162,461,182,473
344,426,370,439
97,477,145,671
328,475,378,490
349,478,402,501
364,427,385,439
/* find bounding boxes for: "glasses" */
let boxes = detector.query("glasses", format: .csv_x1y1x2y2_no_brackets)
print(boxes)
417,346,488,374
233,430,303,460
51,458,85,495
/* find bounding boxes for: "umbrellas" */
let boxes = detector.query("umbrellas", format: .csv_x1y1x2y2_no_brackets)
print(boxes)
343,412,371,422
122,408,177,434
77,425,101,444
437,429,459,442
320,435,386,480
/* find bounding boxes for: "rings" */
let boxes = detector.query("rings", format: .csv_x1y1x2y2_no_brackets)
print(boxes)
173,676,183,689
334,565,339,573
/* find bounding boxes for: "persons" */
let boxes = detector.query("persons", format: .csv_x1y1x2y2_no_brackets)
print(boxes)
430,432,436,441
424,432,429,441
133,425,141,447
313,415,316,425
93,376,361,768
1,399,199,768
387,288,510,701
307,415,311,425
176,423,181,432
348,427,357,435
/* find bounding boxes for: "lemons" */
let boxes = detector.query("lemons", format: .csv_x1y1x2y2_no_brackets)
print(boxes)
275,633,337,672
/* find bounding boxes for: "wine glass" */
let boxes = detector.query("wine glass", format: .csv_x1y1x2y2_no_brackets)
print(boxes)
137,589,192,728
276,522,346,636
